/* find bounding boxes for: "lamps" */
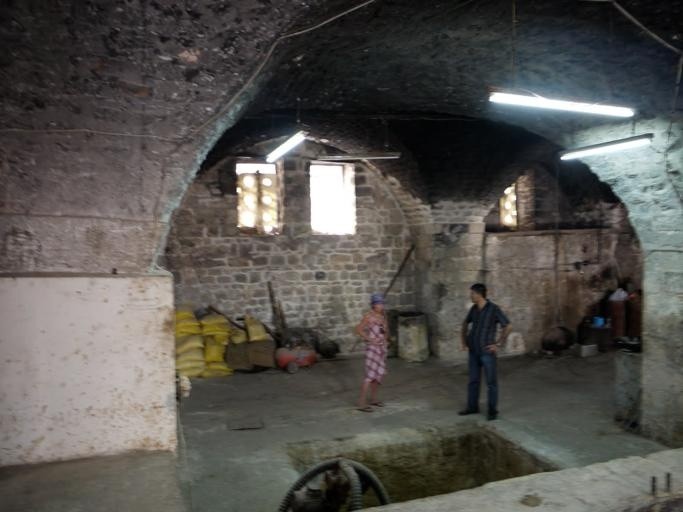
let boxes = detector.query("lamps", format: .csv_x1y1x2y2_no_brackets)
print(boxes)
264,89,309,163
559,114,654,160
315,121,402,161
488,3,636,119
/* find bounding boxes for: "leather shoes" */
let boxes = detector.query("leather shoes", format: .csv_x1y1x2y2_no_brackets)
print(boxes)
459,408,479,415
488,408,498,420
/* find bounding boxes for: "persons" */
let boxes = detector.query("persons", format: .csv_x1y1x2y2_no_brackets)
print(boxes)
457,283,513,421
352,295,391,413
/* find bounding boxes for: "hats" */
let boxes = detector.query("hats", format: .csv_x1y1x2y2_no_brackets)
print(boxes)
371,294,385,304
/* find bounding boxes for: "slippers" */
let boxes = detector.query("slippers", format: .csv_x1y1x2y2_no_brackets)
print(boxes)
357,406,372,412
370,401,384,407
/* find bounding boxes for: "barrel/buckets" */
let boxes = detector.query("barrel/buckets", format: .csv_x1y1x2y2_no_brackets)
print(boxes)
396,311,430,363
383,308,398,357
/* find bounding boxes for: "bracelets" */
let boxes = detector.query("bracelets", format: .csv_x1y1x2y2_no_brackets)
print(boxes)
496,341,501,347
